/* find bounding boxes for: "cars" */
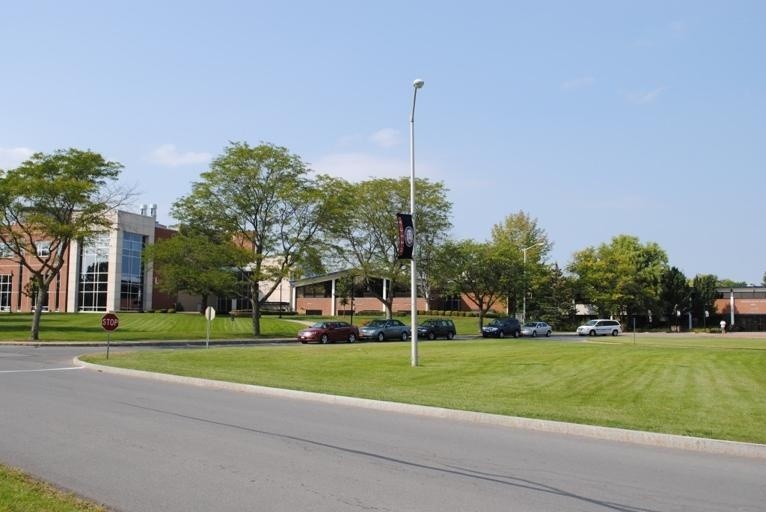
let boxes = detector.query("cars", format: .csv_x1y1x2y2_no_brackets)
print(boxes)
360,319,412,341
521,322,553,337
297,320,358,343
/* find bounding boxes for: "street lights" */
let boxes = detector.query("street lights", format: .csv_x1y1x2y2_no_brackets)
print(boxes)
408,78,423,366
521,242,545,319
351,274,361,324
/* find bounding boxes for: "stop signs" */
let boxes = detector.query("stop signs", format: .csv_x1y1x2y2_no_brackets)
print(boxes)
101,313,118,331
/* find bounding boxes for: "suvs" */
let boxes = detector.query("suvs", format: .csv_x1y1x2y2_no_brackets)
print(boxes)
576,319,623,336
482,316,521,338
417,319,456,340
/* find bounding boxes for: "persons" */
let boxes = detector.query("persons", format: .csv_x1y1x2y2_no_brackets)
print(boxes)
720,319,727,336
